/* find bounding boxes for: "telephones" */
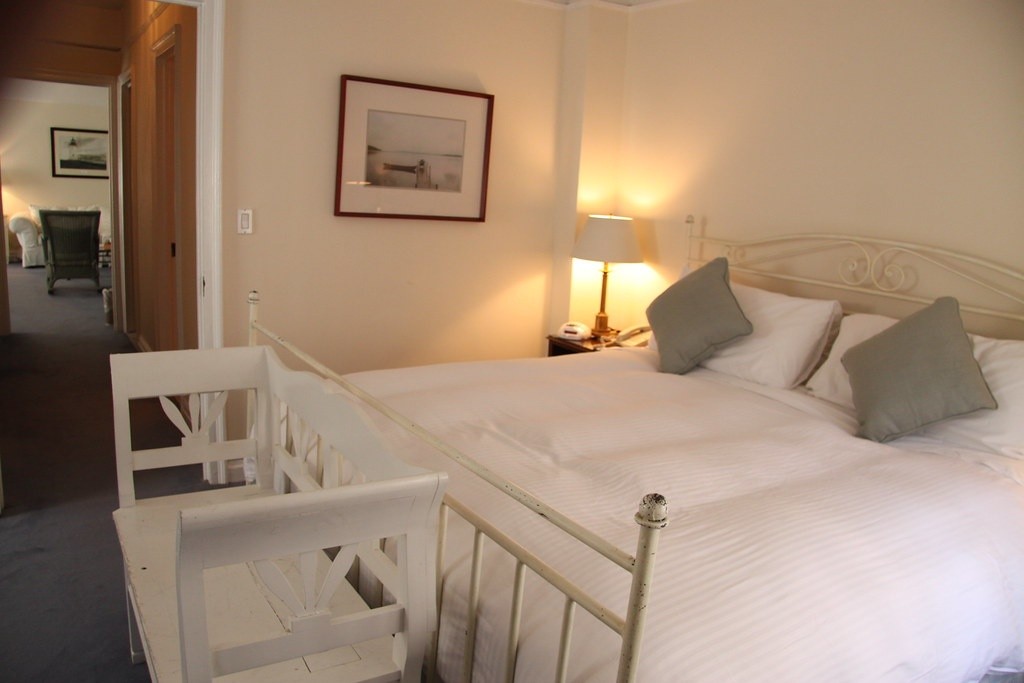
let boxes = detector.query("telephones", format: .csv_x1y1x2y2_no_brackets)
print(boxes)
615,325,653,347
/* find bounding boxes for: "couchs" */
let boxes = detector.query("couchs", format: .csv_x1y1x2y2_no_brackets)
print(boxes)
10,212,112,269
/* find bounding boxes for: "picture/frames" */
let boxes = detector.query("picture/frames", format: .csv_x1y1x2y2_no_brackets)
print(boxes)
333,74,495,222
50,126,110,179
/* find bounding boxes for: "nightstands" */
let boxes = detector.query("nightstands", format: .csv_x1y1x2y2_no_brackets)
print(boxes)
545,333,620,357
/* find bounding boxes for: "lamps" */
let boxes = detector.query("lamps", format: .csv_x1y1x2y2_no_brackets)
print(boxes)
569,215,646,336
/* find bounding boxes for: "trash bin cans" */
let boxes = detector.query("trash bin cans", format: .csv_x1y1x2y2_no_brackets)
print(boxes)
102,288,113,326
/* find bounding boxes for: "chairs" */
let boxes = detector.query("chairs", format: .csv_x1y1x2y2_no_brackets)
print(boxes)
38,210,102,295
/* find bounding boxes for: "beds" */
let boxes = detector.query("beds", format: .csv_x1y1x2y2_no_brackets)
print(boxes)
247,222,1024,683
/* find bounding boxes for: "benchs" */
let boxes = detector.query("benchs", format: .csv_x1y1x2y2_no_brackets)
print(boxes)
110,346,449,683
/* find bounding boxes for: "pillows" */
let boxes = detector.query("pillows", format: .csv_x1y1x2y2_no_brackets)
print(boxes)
644,257,1024,457
29,205,102,234
